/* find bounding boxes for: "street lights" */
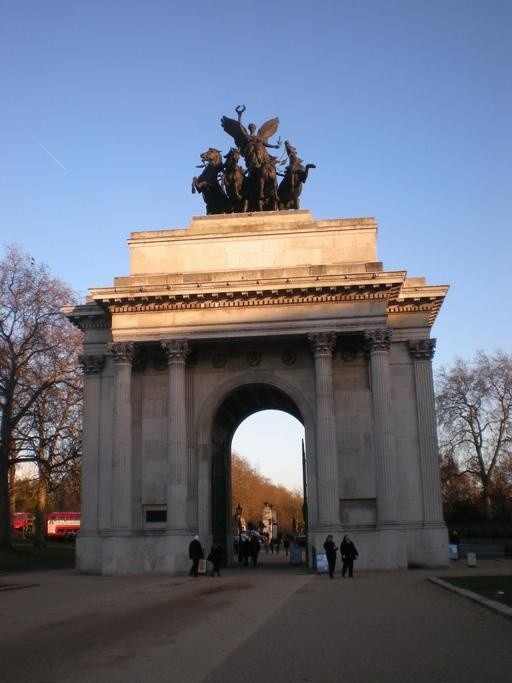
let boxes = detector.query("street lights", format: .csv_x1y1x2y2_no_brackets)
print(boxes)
235,501,244,562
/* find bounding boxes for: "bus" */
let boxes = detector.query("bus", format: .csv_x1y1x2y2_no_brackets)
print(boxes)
14,511,34,536
47,511,80,537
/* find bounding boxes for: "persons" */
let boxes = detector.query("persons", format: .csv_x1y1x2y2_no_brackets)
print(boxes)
207,542,225,577
236,111,281,171
340,534,360,578
188,534,205,577
323,534,339,579
234,535,291,568
